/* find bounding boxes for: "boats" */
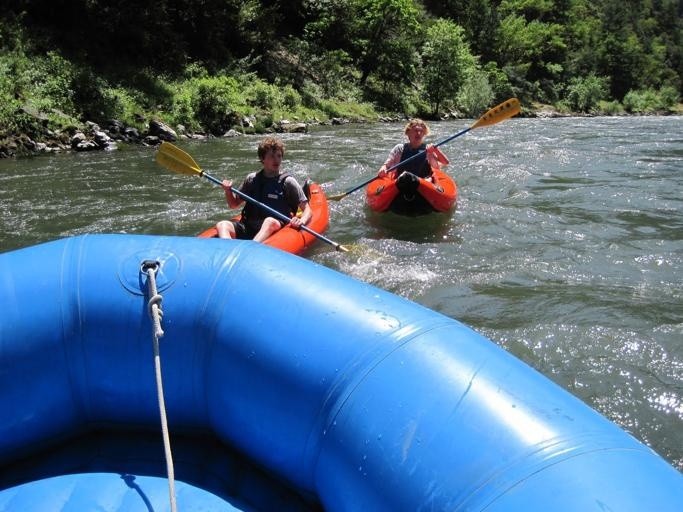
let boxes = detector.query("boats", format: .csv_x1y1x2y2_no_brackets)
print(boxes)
195,168,457,253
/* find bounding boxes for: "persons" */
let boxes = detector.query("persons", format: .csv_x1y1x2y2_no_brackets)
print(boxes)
378,118,449,183
216,137,313,243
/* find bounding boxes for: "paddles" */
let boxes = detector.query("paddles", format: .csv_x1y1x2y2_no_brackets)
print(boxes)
326,97,521,201
156,142,353,253
